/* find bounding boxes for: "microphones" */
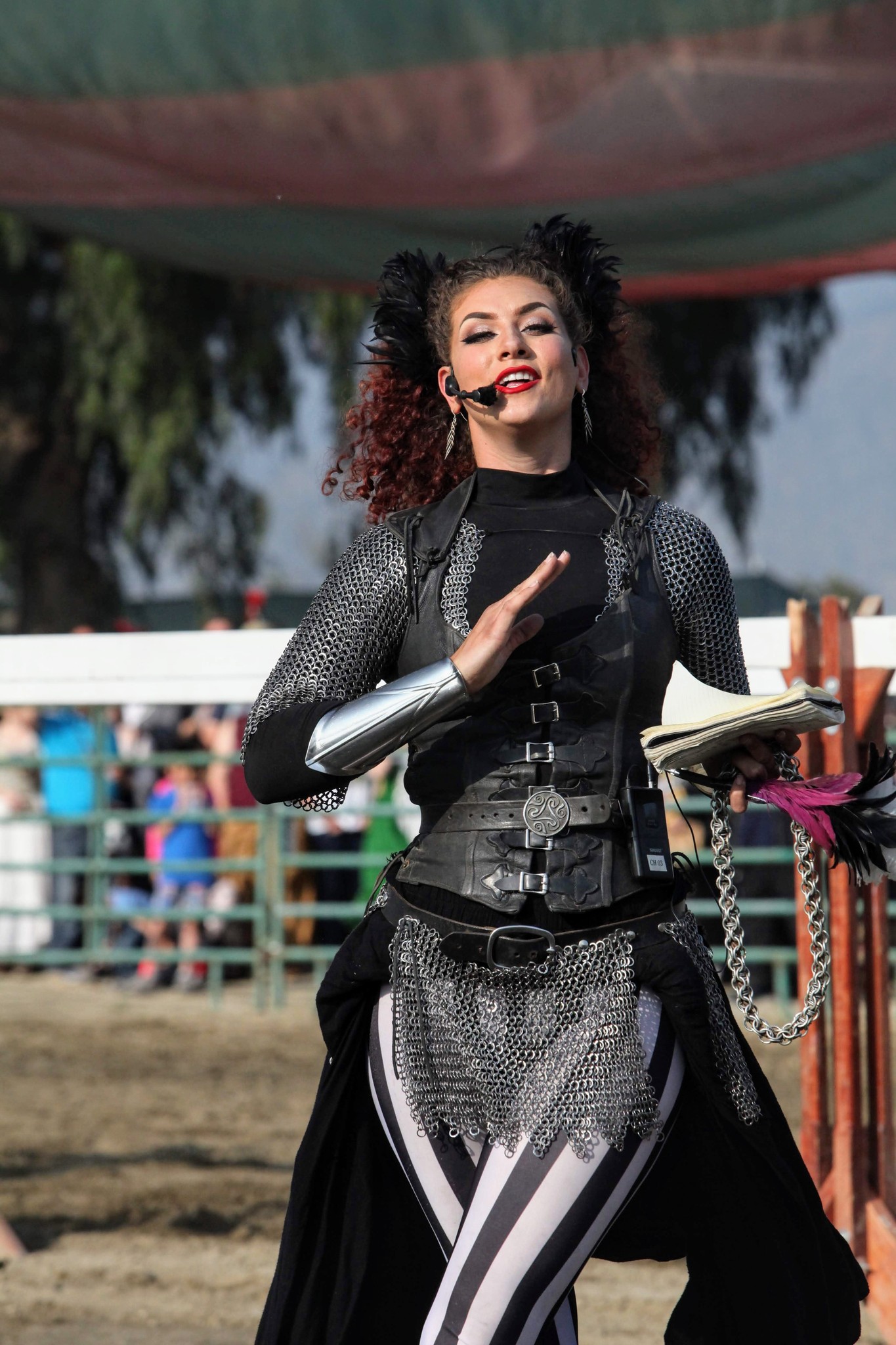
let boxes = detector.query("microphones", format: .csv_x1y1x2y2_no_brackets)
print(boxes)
445,375,497,406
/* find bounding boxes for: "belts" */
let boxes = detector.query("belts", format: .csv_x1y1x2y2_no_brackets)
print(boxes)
432,790,610,836
377,872,667,975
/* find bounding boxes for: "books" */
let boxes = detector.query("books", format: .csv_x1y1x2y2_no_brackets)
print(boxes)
639,660,846,773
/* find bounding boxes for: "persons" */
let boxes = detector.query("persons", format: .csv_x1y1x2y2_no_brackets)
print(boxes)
0,705,420,999
240,212,872,1345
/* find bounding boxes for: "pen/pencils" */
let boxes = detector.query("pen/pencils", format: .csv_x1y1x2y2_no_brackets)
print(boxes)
670,768,766,803
